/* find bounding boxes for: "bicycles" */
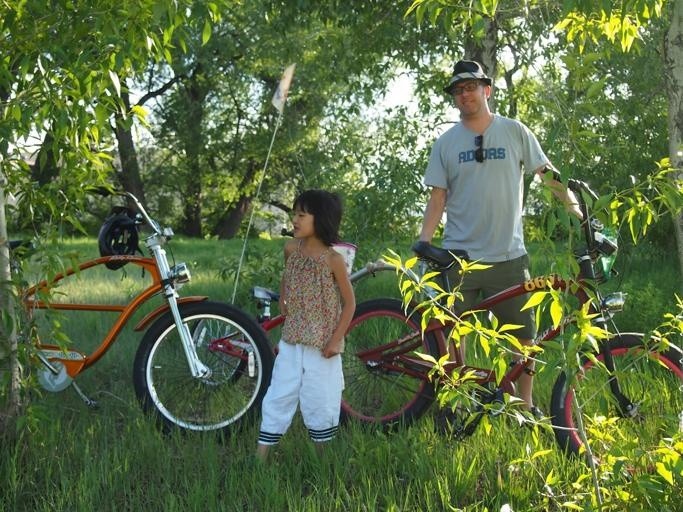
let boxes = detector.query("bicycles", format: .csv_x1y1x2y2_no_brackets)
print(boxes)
339,167,681,474
183,202,351,373
0,183,272,440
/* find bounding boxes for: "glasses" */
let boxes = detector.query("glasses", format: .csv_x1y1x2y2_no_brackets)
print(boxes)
475,136,483,162
451,82,480,95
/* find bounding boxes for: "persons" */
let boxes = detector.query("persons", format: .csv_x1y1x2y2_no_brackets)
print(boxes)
252,190,356,462
418,61,584,433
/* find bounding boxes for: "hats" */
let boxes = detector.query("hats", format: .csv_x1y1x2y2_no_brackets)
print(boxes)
443,61,491,94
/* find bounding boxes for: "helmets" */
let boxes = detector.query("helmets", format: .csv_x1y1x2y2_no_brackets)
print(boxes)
98,216,138,269
